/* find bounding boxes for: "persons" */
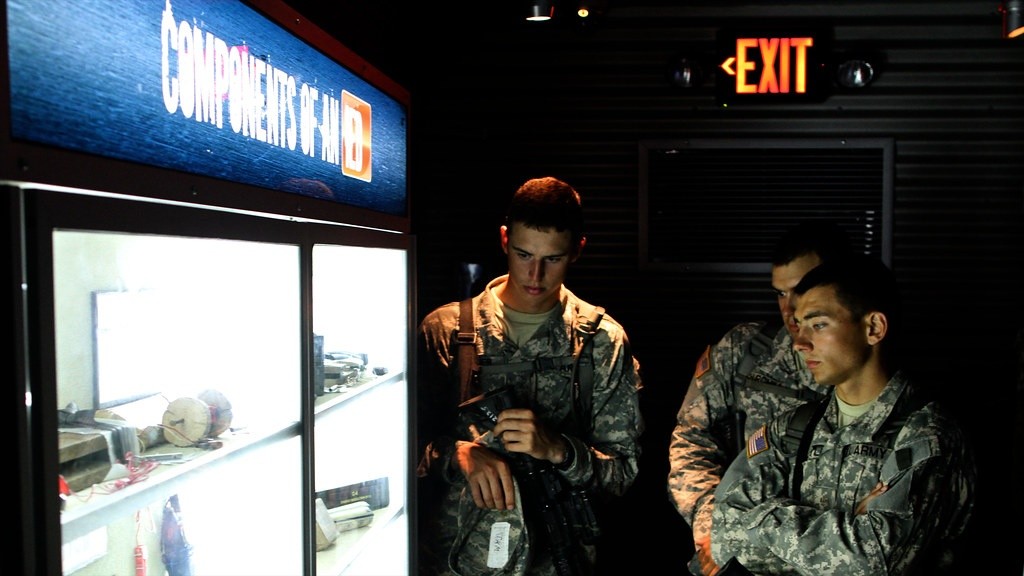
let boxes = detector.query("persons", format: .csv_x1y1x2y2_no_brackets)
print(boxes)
666,222,983,576
417,172,644,576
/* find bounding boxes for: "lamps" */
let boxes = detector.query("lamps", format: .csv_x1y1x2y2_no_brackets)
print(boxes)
1007,0,1024,39
527,0,555,22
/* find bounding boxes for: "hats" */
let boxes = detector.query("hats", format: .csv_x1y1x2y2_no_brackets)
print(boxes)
447,474,531,575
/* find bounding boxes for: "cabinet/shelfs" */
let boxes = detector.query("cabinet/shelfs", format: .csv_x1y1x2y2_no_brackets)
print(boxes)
0,0,421,576
